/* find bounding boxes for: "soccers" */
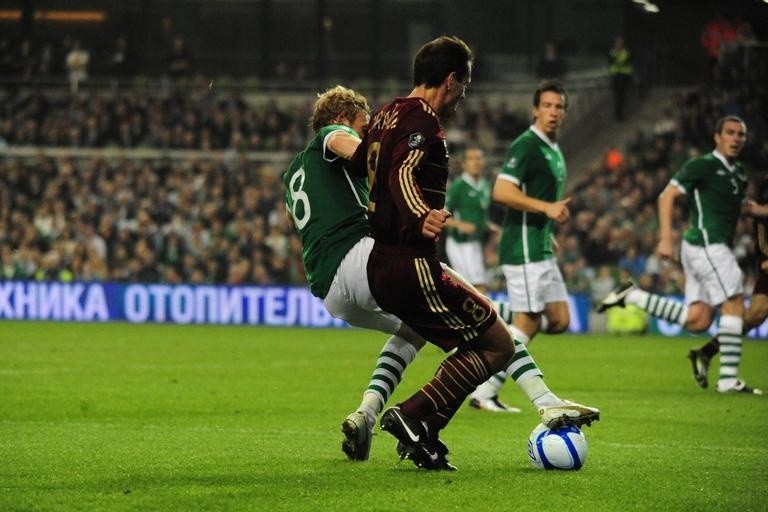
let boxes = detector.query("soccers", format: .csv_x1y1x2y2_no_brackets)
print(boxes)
528,420,588,472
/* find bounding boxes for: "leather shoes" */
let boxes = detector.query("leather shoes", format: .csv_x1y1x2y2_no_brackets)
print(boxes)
536,399,600,430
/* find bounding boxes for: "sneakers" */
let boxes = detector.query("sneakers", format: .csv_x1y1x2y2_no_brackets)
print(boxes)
716,377,763,397
596,278,636,316
687,348,708,388
396,439,459,472
468,395,521,415
341,409,373,461
379,402,438,461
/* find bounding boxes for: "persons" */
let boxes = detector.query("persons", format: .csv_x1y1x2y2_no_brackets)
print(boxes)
681,172,768,388
363,34,516,472
0,0,768,295
571,111,762,397
470,85,572,417
445,145,500,294
278,84,602,460
591,111,762,397
